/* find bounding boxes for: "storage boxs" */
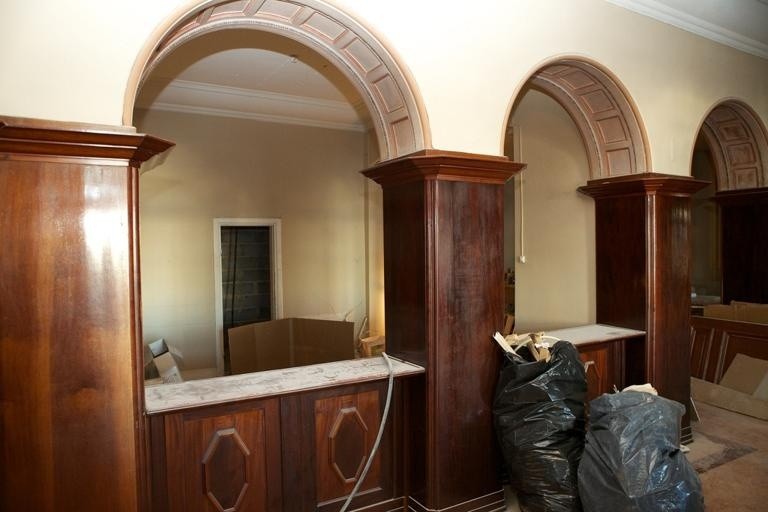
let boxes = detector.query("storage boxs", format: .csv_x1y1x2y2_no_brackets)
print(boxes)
143,338,183,384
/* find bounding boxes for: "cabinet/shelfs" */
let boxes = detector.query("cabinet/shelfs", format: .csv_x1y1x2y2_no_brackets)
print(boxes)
575,340,624,422
149,377,408,512
